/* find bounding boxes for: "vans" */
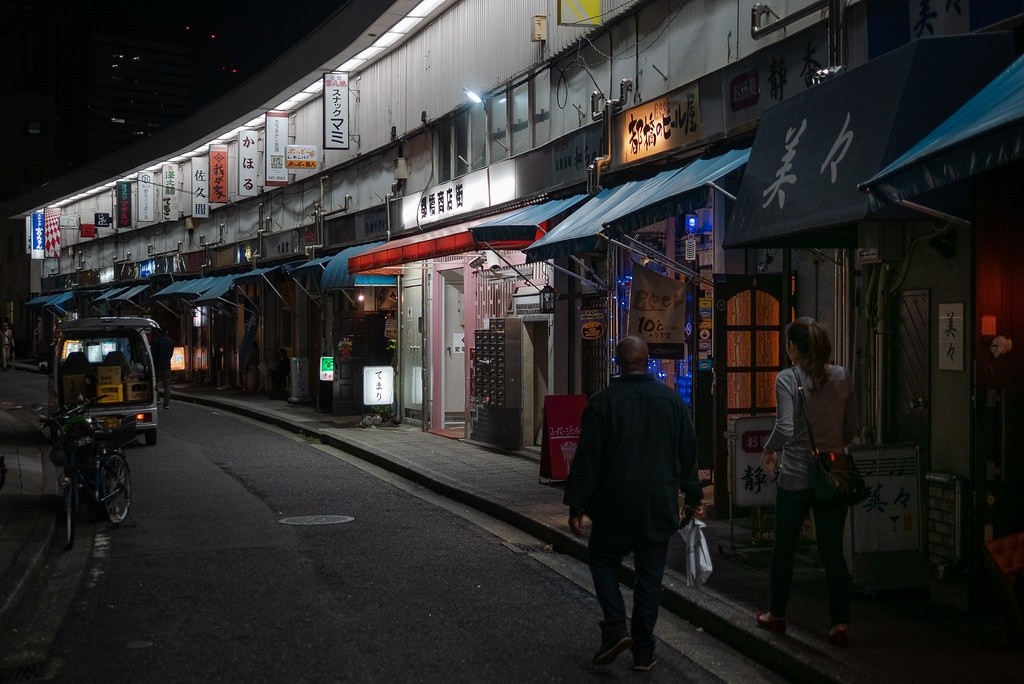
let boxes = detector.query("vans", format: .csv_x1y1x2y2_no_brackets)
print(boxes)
55,316,161,446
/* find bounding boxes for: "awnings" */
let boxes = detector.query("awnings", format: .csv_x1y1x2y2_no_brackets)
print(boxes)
521,146,752,263
722,29,1017,248
150,242,396,307
858,54,1024,217
90,285,149,306
348,194,587,275
25,291,73,317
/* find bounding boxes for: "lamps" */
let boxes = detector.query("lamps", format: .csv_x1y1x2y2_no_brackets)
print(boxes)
539,257,556,314
461,86,488,112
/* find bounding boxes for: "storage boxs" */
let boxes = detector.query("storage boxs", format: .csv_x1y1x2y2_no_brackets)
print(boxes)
62,362,150,404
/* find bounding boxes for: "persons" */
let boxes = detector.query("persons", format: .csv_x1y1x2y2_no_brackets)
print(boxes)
151,325,174,408
564,336,704,670
0,317,16,371
757,318,854,648
278,348,290,376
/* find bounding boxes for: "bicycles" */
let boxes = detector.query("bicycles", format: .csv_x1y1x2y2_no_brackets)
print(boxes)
53,442,137,550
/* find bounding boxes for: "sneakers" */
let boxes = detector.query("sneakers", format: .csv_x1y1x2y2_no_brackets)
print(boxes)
156,400,160,405
823,627,850,647
633,652,657,670
754,609,790,634
592,626,631,663
163,404,169,409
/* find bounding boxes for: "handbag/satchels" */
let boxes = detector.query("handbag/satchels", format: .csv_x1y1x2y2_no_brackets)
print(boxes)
679,517,713,590
810,448,870,510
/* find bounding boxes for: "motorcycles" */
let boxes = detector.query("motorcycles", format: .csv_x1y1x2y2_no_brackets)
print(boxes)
39,395,115,469
